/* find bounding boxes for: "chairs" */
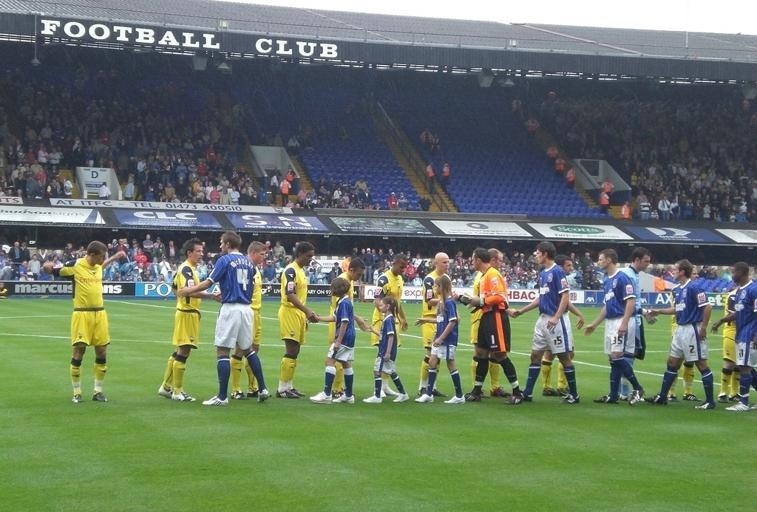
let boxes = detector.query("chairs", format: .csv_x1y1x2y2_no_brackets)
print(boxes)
427,161,614,218
292,87,387,147
403,125,544,161
368,182,423,210
298,147,408,182
389,83,519,124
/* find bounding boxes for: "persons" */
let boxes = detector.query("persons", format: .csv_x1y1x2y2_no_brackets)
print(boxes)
508,240,581,406
276,241,320,400
369,255,408,395
179,230,273,406
461,247,521,404
646,258,716,411
328,257,367,402
541,252,584,397
439,162,452,197
469,248,512,399
586,248,645,404
41,240,126,403
431,132,443,153
566,251,601,290
362,296,409,403
197,240,319,285
127,233,185,284
718,266,754,404
420,126,432,147
327,247,539,289
413,275,466,404
43,240,126,280
229,239,267,399
648,263,757,281
0,49,426,206
157,238,219,402
512,73,756,218
0,239,41,282
417,252,457,398
710,261,757,411
426,161,439,199
617,247,658,402
664,264,703,403
309,277,357,404
